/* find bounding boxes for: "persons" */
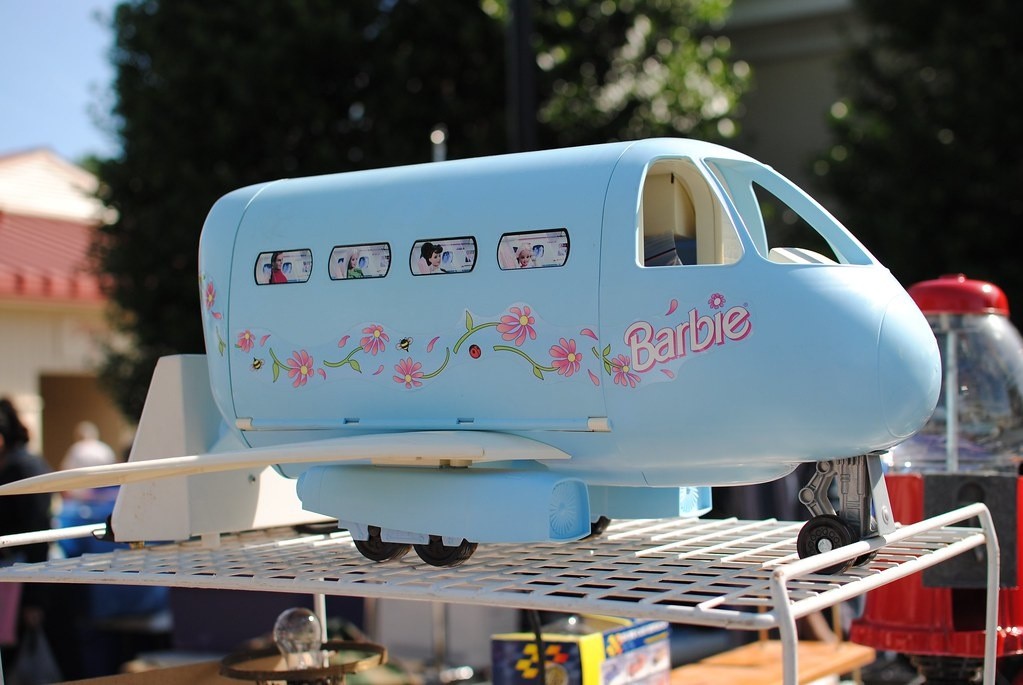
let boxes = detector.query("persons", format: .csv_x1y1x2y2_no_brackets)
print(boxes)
56,440,166,677
0,398,51,685
729,472,798,521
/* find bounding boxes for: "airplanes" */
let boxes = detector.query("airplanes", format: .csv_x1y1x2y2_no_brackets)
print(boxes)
0,138,942,569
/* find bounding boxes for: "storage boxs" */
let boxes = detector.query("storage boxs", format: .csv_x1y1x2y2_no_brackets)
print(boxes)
488,613,671,685
